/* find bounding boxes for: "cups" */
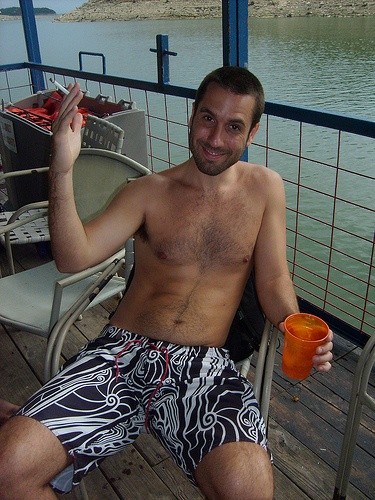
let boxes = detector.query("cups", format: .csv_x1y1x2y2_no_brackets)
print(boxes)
281,312,329,380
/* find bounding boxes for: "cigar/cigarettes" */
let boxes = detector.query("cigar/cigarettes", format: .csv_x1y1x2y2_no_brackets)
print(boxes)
49,78,70,96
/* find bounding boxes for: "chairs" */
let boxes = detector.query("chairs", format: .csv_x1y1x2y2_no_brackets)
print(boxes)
328,334,375,500
0,114,279,473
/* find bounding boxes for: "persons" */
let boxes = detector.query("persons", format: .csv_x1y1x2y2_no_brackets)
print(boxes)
0,66,333,500
